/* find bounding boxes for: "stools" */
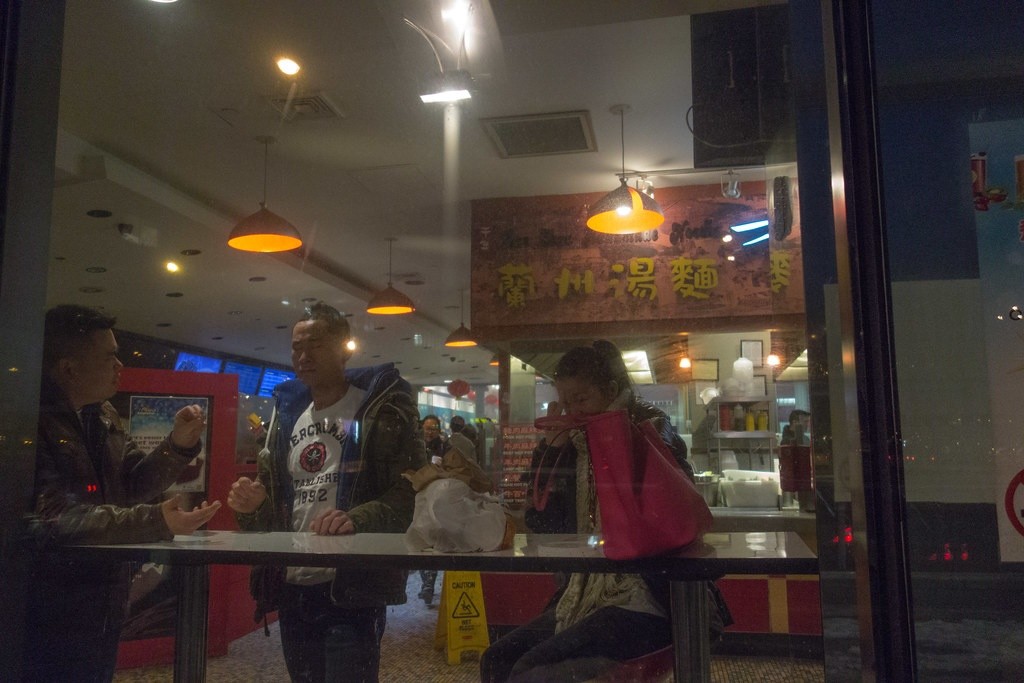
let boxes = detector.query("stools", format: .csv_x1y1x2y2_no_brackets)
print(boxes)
594,641,675,683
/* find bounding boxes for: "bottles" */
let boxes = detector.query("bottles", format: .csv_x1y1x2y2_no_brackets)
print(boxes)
719,403,767,431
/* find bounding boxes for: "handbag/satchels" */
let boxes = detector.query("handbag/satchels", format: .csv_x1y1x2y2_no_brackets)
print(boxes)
532,409,715,560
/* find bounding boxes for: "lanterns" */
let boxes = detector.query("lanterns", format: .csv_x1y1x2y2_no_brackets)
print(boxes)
448,380,497,407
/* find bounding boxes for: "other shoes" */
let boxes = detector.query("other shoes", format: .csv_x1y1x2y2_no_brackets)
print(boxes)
418,589,433,604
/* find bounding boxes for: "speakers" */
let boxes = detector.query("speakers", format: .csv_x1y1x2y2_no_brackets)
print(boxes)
758,4,799,166
692,6,758,169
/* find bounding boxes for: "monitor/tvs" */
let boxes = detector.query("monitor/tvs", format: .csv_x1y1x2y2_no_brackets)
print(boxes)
220,358,264,396
255,363,298,396
171,349,224,373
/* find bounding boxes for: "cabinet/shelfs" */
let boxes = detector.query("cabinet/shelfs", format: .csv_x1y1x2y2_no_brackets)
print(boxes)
704,396,777,506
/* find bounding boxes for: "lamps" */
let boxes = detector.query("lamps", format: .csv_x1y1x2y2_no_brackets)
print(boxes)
445,289,478,347
720,170,742,199
488,353,499,366
420,69,473,105
228,137,301,252
638,171,655,197
366,236,415,313
679,338,691,367
586,103,666,235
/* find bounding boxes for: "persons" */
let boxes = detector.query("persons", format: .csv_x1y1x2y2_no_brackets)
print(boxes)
226,299,427,683
14,303,221,682
480,340,697,682
403,413,501,604
776,410,813,503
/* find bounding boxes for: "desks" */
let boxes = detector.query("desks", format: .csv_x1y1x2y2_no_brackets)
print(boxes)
67,528,821,683
